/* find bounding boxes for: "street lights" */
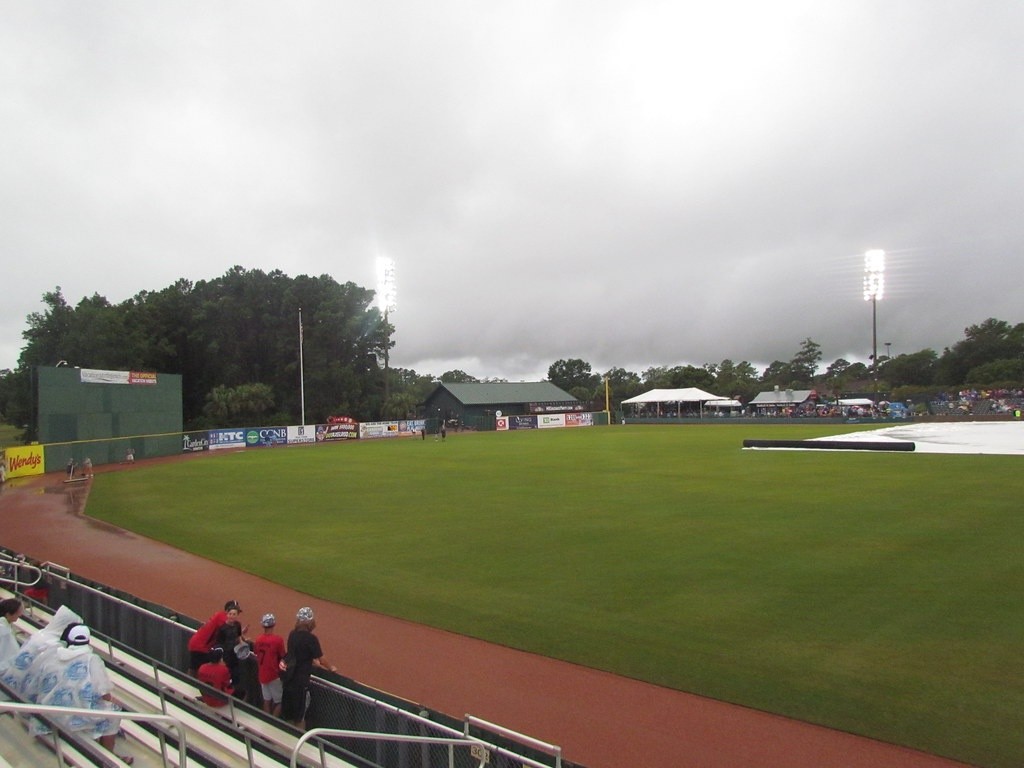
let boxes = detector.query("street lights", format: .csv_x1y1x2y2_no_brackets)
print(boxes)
379,257,398,421
862,249,886,392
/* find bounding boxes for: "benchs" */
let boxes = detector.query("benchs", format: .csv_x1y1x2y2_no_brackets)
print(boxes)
0,586,358,768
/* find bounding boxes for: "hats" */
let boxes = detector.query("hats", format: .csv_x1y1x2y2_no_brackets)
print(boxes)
260,614,276,626
296,607,318,622
225,600,243,614
68,625,90,642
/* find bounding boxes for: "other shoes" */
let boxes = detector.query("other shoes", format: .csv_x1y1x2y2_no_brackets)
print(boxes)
122,756,133,765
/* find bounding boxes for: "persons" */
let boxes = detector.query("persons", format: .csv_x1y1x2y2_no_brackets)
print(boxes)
2,610,134,768
67,456,94,477
6,554,50,607
0,598,24,662
126,448,136,464
421,426,446,443
254,613,286,718
189,600,251,711
282,606,337,733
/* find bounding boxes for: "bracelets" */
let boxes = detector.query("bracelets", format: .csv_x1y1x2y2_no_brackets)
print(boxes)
329,665,337,672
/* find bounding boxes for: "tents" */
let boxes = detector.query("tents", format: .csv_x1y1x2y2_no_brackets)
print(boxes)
704,400,742,413
620,387,731,419
831,398,874,408
